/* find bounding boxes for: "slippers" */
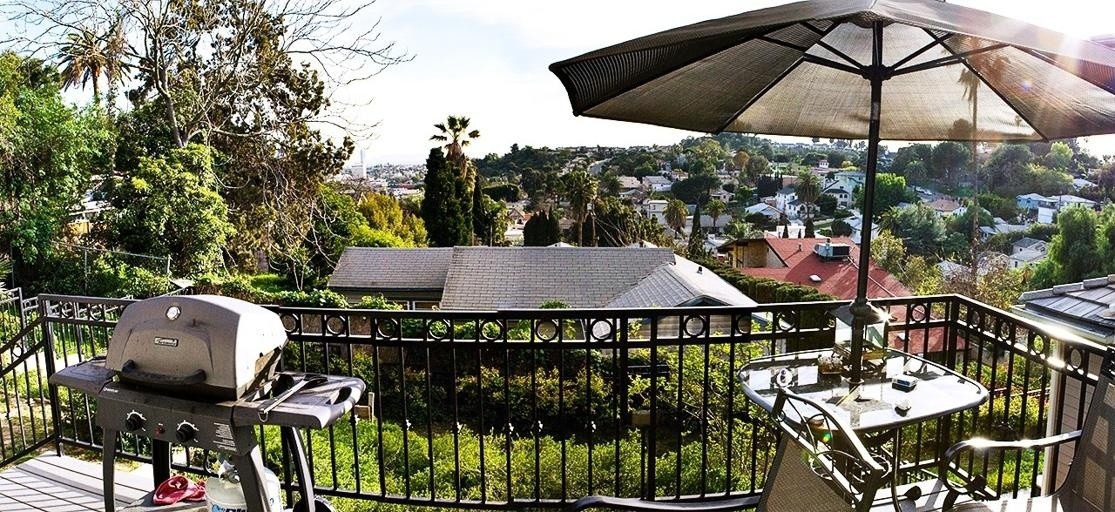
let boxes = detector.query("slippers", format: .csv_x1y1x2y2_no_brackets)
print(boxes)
152,473,207,506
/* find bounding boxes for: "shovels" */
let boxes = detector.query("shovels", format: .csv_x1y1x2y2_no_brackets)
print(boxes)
256,373,327,414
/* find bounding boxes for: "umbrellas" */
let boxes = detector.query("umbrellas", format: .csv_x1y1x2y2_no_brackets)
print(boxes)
548,0,1115,392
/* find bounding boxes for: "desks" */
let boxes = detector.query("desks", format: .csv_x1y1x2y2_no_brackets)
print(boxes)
740,346,989,502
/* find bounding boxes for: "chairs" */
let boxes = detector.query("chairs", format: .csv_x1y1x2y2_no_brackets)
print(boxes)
561,385,884,512
939,345,1115,511
